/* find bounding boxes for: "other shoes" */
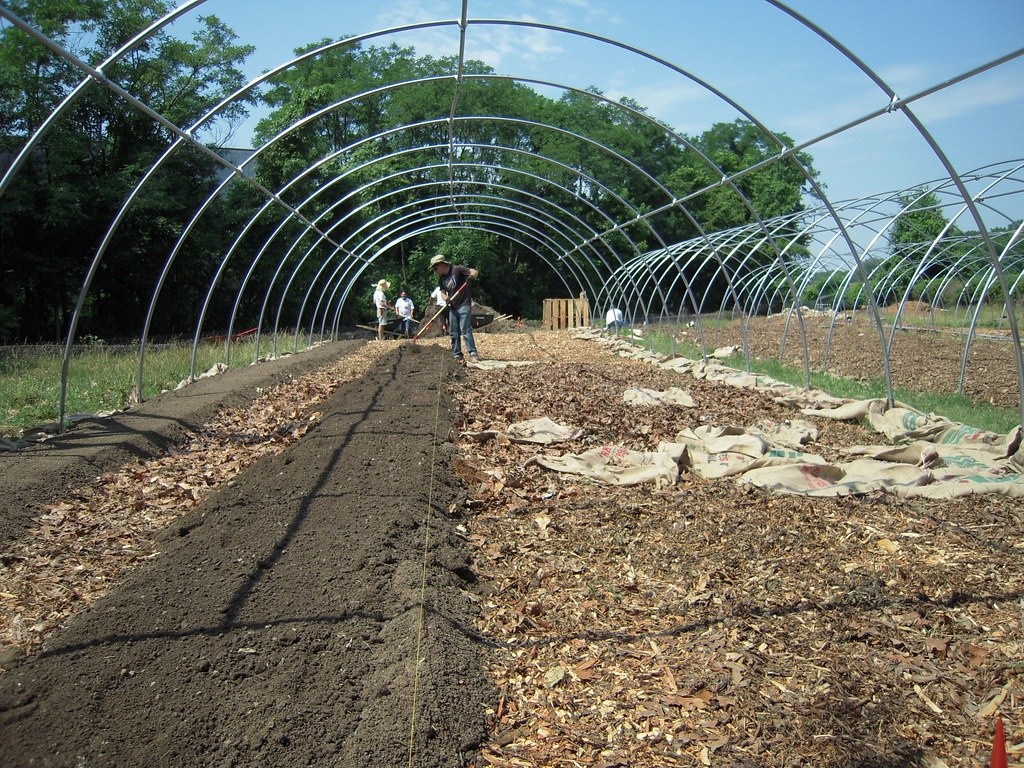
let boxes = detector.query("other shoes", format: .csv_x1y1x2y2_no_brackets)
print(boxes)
471,351,478,356
454,355,462,359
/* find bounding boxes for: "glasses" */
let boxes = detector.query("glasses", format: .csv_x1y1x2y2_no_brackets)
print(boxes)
434,264,441,270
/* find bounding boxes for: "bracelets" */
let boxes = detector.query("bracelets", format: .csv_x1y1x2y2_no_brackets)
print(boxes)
471,275,474,279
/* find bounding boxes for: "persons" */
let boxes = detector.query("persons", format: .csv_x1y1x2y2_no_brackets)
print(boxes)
394,290,414,339
373,280,391,340
607,304,623,329
428,255,478,360
429,287,449,336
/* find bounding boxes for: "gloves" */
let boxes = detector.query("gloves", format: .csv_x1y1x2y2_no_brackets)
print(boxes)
465,275,474,283
446,298,454,307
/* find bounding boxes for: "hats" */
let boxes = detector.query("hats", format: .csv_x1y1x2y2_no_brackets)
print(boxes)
377,279,391,291
428,255,452,269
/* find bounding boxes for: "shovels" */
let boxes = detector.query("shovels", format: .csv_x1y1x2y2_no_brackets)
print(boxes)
401,283,469,350
